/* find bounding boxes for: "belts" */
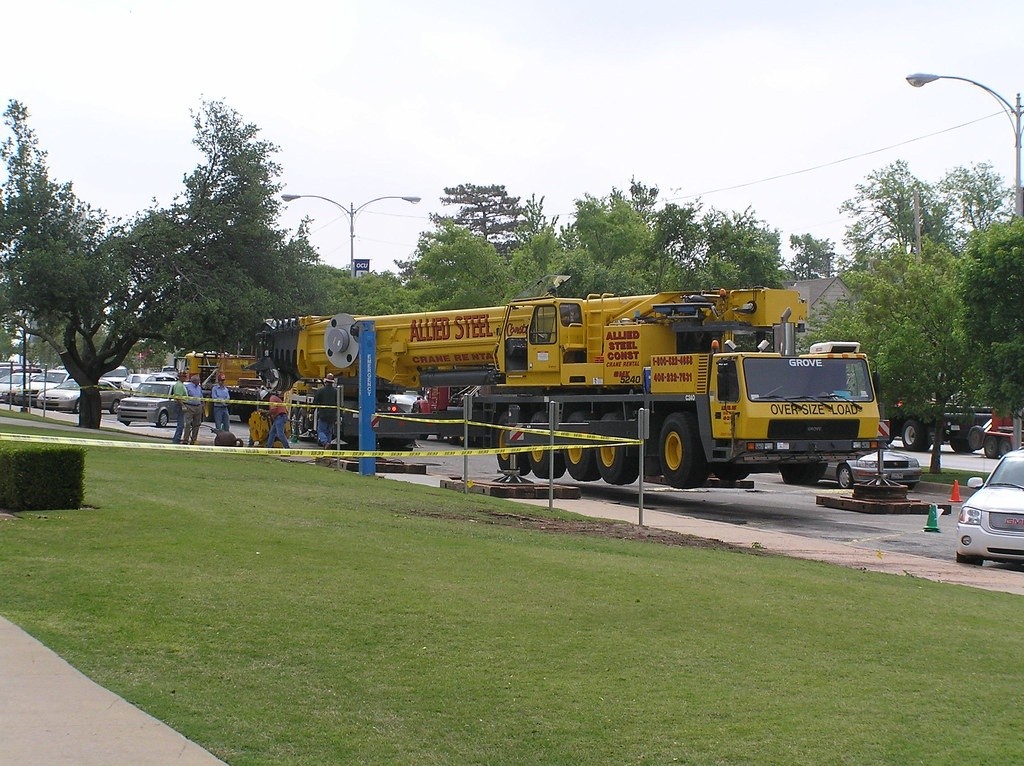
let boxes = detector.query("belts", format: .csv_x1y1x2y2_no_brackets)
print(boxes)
187,404,201,406
174,401,188,404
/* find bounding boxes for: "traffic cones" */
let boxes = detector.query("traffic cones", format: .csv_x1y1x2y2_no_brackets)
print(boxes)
922,501,940,532
0,361,180,405
947,480,963,502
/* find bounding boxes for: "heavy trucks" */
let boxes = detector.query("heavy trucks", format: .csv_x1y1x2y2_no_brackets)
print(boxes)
878,397,1024,459
172,351,442,451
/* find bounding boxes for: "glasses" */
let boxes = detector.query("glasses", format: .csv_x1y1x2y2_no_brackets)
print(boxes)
183,375,187,377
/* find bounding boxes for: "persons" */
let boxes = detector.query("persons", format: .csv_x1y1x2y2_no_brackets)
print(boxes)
182,374,205,445
260,390,290,449
172,371,189,444
212,373,230,432
309,373,343,450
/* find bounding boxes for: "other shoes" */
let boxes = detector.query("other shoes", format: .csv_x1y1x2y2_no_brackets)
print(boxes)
323,442,332,450
183,442,188,445
190,443,194,445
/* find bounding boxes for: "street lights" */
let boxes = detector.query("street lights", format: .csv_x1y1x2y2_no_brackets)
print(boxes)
282,194,422,276
907,72,1024,219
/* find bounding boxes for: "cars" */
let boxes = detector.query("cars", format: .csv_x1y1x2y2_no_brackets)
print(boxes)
117,381,192,428
820,441,922,490
36,379,126,416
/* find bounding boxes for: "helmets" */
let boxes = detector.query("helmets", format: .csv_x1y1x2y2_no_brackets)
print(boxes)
260,389,268,400
326,373,334,383
218,373,226,381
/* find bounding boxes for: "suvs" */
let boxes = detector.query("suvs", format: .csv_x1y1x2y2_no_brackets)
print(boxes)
955,446,1024,567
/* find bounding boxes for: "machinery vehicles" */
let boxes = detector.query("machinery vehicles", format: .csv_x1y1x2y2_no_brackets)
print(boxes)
252,274,881,490
